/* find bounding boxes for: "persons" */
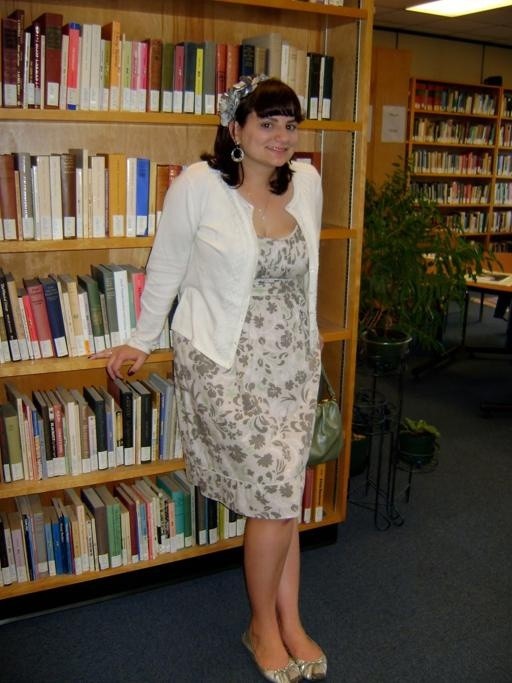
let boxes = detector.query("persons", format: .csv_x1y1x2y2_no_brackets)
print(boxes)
88,74,329,683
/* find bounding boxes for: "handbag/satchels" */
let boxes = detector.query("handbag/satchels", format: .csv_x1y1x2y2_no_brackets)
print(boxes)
306,360,345,466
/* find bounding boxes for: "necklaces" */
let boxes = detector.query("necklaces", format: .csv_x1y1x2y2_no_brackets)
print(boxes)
237,179,279,221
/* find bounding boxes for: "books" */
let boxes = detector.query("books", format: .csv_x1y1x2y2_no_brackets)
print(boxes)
1,470,247,585
1,373,184,484
303,462,326,523
465,271,510,284
0,148,186,242
293,151,321,175
0,9,334,121
410,84,512,253
1,263,180,363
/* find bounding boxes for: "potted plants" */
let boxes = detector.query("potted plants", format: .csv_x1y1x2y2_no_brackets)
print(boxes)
394,416,438,466
355,152,493,369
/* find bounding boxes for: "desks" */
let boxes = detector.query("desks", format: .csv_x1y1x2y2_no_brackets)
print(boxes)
355,326,449,532
422,260,511,351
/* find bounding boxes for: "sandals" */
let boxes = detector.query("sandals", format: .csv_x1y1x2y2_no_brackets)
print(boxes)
243,625,329,683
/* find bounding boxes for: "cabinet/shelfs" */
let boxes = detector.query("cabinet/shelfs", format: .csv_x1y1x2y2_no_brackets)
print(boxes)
366,48,512,251
0,0,375,614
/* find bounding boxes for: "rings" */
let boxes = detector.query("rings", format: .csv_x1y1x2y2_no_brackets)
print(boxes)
110,348,114,356
